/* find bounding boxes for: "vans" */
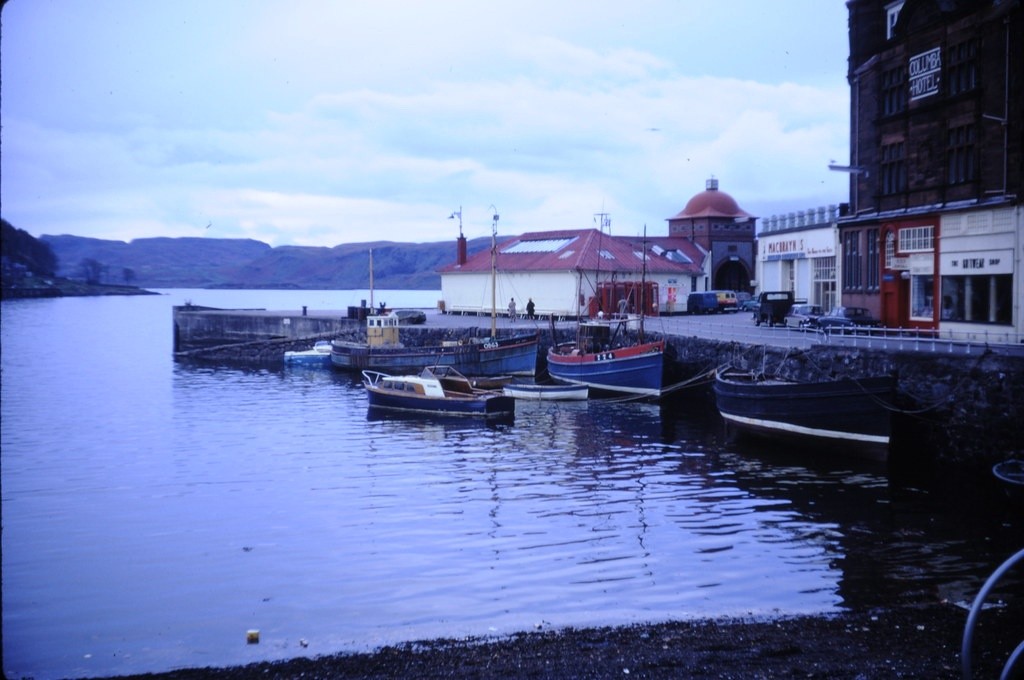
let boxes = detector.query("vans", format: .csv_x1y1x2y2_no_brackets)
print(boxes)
686,289,760,316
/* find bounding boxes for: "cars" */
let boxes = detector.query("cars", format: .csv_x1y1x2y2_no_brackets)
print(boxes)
816,306,878,334
783,304,826,333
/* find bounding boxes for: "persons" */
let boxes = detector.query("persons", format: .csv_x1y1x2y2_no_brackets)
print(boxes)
527,299,535,320
508,298,517,322
617,295,630,325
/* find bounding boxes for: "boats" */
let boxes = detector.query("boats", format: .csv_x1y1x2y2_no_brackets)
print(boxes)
361,369,515,423
501,383,588,401
712,362,897,446
547,263,665,396
283,338,332,370
331,246,540,379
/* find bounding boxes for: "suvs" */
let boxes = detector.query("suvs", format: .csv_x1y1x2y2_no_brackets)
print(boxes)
751,290,796,327
